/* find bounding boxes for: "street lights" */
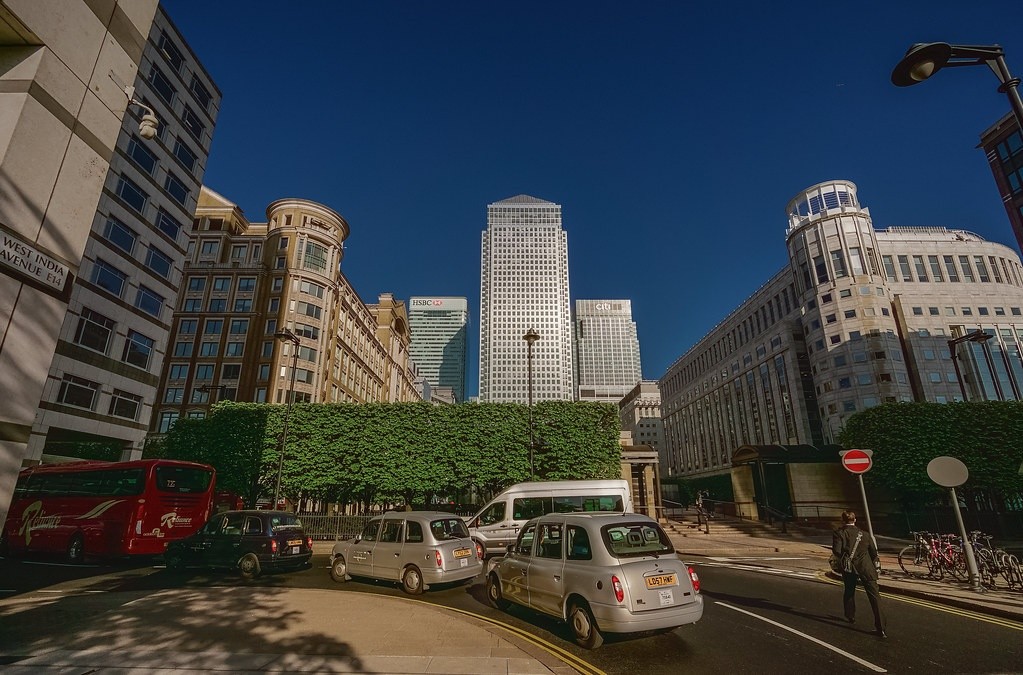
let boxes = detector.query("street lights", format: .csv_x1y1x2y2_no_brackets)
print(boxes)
273,327,300,510
522,328,540,481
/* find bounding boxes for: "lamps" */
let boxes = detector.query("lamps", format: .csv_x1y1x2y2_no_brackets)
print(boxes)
123,84,159,141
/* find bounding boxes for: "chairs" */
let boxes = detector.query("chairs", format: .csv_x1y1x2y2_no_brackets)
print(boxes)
609,528,670,553
541,528,561,557
223,518,243,536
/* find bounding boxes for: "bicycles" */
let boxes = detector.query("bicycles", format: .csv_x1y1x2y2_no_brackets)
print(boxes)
925,533,967,580
898,530,935,578
978,533,1014,582
951,534,997,578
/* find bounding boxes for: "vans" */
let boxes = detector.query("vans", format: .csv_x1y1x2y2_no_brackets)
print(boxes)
465,479,634,560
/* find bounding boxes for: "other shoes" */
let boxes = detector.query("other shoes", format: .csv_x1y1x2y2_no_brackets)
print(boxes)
876,629,887,638
848,617,856,624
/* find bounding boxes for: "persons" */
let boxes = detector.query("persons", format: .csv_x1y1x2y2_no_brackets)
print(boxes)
833,511,888,639
696,494,710,534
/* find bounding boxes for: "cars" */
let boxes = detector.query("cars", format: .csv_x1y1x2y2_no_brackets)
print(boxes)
165,510,313,577
484,511,703,649
327,511,483,595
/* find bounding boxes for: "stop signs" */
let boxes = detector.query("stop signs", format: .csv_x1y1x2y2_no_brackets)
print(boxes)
842,449,872,473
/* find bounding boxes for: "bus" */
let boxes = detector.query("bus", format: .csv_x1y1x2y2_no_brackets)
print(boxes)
0,460,216,563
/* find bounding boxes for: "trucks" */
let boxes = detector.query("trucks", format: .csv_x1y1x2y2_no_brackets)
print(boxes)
217,490,286,511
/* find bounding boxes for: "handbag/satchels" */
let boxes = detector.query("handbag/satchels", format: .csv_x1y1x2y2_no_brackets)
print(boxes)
828,551,859,577
